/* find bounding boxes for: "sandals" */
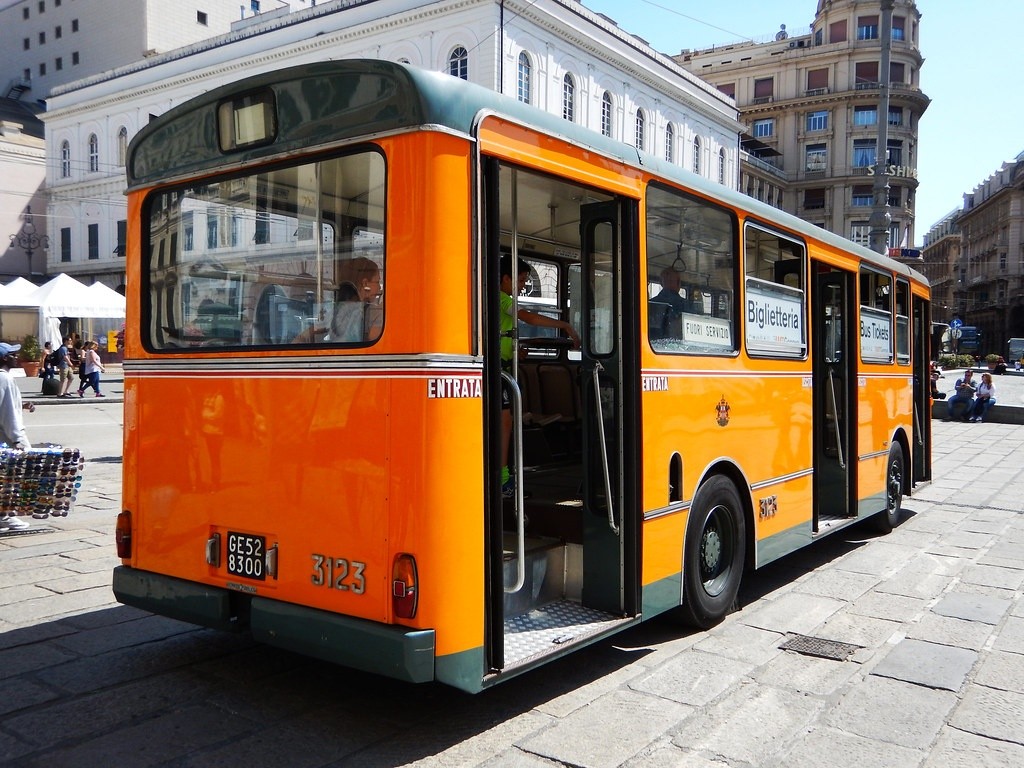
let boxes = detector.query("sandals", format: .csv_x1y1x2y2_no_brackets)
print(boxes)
64,393,71,396
57,394,66,397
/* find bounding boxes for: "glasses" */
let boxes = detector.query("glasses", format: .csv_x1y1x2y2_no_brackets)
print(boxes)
8,353,17,356
1,449,85,520
965,374,970,376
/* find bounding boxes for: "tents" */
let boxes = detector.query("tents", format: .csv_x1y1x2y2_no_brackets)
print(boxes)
0,275,126,351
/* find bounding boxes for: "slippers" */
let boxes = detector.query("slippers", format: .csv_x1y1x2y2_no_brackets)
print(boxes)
96,394,104,397
78,389,84,398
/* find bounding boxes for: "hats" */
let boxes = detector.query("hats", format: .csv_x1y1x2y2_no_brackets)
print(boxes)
1,342,22,355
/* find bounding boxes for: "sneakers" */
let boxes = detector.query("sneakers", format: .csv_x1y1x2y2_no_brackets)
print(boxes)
501,466,508,485
969,417,974,421
976,417,982,421
1,517,31,535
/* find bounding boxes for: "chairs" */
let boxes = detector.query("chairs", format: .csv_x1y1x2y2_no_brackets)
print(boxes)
198,297,583,499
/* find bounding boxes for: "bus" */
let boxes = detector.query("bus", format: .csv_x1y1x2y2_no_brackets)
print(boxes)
113,60,932,693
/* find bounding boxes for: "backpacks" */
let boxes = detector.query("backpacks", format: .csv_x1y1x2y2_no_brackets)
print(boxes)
49,349,59,366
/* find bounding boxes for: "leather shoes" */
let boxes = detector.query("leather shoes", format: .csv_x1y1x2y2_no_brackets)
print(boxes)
942,416,954,420
959,416,968,421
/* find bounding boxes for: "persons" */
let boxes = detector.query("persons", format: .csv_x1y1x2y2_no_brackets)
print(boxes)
929,362,946,399
42,342,54,395
0,342,35,532
969,373,997,423
77,341,106,398
312,257,384,343
943,371,979,423
49,337,75,399
648,266,704,343
498,253,579,500
764,267,774,282
994,357,1007,374
